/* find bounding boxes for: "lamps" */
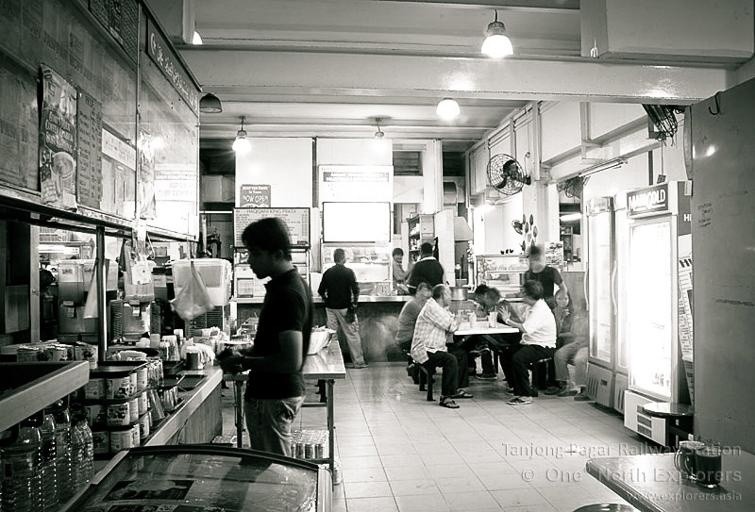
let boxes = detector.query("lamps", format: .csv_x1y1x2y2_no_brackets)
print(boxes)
481,10,514,62
372,117,386,142
437,97,459,121
197,91,221,113
230,115,250,153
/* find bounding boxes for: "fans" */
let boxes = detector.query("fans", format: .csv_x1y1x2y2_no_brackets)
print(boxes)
641,104,685,139
486,154,531,196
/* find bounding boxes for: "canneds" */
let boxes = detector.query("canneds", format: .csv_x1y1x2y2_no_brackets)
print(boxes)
16,341,153,453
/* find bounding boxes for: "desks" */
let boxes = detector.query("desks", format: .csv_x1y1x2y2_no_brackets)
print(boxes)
585,448,755,512
221,333,346,487
448,320,520,354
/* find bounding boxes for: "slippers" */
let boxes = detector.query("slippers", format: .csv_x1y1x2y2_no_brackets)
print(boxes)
507,395,533,404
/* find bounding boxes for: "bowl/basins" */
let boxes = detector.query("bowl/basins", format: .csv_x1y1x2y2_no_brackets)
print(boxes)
224,314,338,357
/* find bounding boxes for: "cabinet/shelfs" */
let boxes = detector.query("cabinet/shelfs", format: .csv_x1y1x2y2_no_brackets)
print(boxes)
477,254,530,302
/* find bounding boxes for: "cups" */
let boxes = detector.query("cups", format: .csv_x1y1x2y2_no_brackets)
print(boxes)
457,309,464,322
487,315,496,329
467,313,477,329
490,311,499,327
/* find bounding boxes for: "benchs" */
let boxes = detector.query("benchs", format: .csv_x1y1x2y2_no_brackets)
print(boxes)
403,346,436,402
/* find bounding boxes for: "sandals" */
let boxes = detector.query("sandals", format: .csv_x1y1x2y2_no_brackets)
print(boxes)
451,390,472,398
440,396,459,408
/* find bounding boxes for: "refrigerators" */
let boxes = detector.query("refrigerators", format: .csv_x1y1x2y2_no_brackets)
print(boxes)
585,213,618,371
622,214,678,403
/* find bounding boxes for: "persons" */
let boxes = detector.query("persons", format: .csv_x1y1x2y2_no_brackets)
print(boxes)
317,248,368,369
214,217,313,461
393,242,591,410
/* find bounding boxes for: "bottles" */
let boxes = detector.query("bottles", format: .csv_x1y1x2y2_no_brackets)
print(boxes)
653,371,671,388
0,403,97,510
408,236,419,263
477,259,528,282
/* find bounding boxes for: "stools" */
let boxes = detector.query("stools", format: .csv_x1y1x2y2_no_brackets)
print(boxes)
574,502,633,511
643,402,694,454
